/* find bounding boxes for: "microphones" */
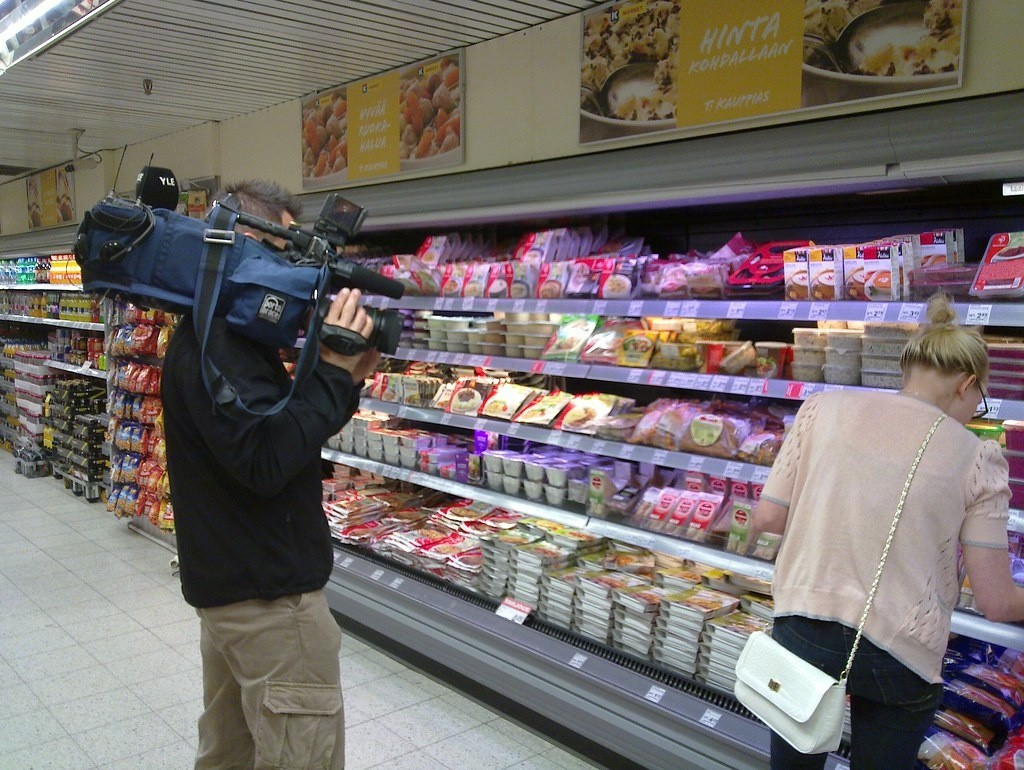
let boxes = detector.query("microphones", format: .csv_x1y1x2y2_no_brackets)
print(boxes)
335,261,405,300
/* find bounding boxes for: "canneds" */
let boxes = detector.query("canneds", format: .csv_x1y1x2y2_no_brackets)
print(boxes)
70,335,107,370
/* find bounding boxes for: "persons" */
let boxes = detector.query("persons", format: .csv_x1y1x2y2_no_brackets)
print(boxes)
751,292,1024,770
160,179,380,770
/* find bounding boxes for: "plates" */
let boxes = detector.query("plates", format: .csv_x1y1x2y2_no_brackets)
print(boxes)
401,145,460,171
802,61,959,88
580,106,676,128
302,165,348,185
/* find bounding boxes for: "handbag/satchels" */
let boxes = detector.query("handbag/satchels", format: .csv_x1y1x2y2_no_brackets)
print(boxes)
733,630,847,756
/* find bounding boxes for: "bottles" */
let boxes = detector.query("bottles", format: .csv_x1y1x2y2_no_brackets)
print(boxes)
0,350,78,455
0,254,82,285
0,290,109,323
13,442,50,479
43,375,111,504
1,326,71,364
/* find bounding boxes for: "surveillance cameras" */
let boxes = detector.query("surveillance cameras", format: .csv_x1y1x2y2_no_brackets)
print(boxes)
65,157,97,172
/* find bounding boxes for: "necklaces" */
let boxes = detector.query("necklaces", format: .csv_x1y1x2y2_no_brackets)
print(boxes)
902,390,918,396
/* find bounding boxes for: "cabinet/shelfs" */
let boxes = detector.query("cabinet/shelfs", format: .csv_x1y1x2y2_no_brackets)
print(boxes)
0,163,1023,770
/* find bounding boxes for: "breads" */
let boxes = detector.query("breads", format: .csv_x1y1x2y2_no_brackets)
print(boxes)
632,398,788,468
917,647,1024,770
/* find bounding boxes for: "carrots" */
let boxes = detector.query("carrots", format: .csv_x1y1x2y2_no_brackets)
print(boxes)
398,66,460,159
302,99,350,176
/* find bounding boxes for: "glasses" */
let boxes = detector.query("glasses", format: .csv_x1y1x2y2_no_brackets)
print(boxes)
971,380,989,420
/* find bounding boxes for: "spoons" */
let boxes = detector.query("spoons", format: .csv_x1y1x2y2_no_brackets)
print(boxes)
581,62,658,119
803,2,932,75
